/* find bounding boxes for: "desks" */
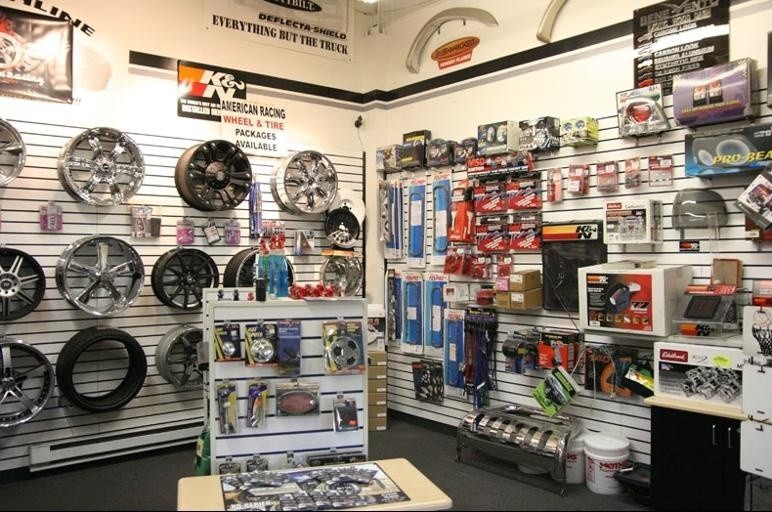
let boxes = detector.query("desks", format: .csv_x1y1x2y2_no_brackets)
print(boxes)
176,456,454,512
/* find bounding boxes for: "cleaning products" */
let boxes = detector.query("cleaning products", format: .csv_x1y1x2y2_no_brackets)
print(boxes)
254,233,289,297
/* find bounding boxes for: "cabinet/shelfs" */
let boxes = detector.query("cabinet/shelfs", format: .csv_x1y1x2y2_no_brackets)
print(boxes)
648,404,747,512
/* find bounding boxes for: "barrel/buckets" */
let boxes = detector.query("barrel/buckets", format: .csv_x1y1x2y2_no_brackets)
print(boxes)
552,433,584,484
518,424,549,477
584,433,630,494
